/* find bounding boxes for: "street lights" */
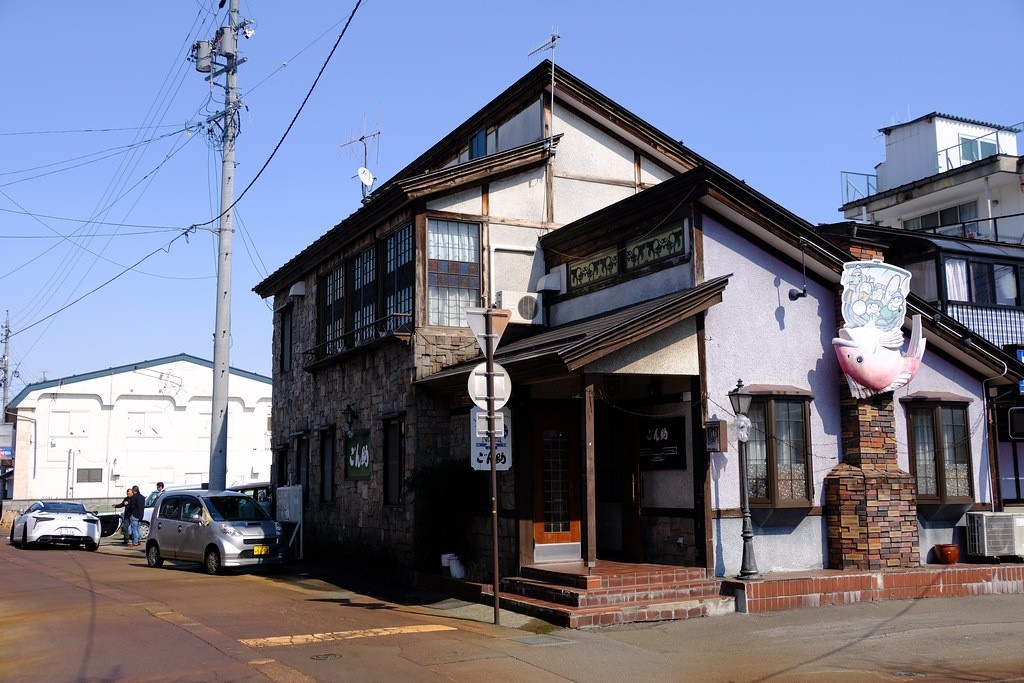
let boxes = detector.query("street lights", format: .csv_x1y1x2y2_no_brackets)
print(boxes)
723,376,760,580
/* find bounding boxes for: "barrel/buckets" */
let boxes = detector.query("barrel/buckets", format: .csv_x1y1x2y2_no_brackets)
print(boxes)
441,553,455,566
448,555,465,579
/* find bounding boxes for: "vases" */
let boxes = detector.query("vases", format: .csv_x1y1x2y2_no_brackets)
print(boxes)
935,543,959,564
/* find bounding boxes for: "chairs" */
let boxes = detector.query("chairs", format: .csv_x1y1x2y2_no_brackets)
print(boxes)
173,501,186,512
189,502,202,516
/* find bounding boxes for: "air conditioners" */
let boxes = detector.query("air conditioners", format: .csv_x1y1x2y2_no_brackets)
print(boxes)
966,512,1024,564
497,291,542,324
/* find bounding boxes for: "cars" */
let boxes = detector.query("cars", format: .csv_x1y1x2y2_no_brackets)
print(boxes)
9,500,124,552
120,481,278,575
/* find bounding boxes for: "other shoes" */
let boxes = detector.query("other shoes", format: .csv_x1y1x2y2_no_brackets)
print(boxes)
121,542,128,544
131,544,138,546
138,541,141,545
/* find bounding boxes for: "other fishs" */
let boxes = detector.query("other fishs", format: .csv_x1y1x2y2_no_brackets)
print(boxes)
832,314,927,399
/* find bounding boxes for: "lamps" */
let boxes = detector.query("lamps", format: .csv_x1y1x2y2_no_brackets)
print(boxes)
933,313,973,347
789,244,808,301
341,404,358,431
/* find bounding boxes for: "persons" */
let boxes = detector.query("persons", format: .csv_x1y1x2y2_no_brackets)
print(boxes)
112,489,133,544
128,486,145,546
153,482,166,507
